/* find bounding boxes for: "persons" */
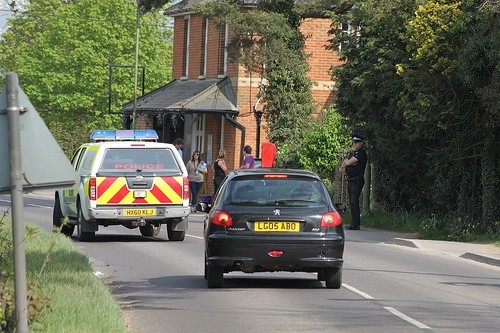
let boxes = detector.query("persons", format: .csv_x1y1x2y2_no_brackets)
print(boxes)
213,148,228,203
172,137,186,161
240,145,255,174
185,150,208,210
339,134,369,231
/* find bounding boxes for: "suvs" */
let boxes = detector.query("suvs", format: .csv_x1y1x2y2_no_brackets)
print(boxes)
52,129,190,241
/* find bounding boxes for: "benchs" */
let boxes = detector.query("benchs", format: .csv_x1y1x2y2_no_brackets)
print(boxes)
235,185,314,201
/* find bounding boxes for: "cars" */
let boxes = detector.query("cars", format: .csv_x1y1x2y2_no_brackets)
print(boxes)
195,166,348,289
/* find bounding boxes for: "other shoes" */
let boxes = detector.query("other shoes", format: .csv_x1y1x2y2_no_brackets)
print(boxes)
191,207,196,212
347,225,360,230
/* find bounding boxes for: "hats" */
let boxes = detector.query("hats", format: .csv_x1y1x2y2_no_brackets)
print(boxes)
352,134,365,143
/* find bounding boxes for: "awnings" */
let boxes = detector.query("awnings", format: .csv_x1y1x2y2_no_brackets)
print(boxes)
121,78,239,113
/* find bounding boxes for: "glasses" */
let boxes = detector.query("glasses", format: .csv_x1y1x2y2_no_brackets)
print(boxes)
194,154,198,155
